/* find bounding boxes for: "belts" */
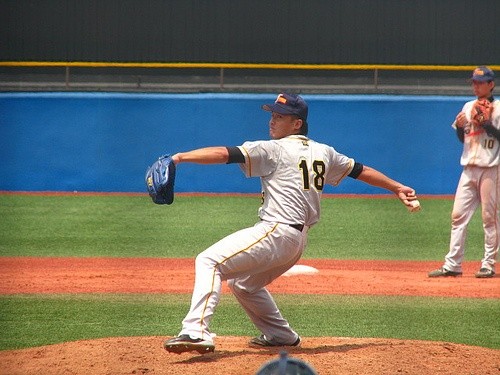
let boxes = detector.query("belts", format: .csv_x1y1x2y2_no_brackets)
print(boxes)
289,224,311,233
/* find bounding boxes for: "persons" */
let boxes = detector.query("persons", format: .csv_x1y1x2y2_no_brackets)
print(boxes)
145,94,420,354
428,66,500,279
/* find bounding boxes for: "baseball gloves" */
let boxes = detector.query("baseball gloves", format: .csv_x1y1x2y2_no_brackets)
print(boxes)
470,97,492,125
141,152,175,204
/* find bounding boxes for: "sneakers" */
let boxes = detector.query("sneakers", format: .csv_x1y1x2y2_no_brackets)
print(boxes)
248,333,302,347
475,268,495,278
428,267,462,278
163,334,215,354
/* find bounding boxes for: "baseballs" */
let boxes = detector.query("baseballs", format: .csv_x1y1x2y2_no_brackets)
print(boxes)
409,199,420,208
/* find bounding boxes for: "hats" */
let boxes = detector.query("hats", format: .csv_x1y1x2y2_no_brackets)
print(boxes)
262,91,308,120
468,66,494,82
